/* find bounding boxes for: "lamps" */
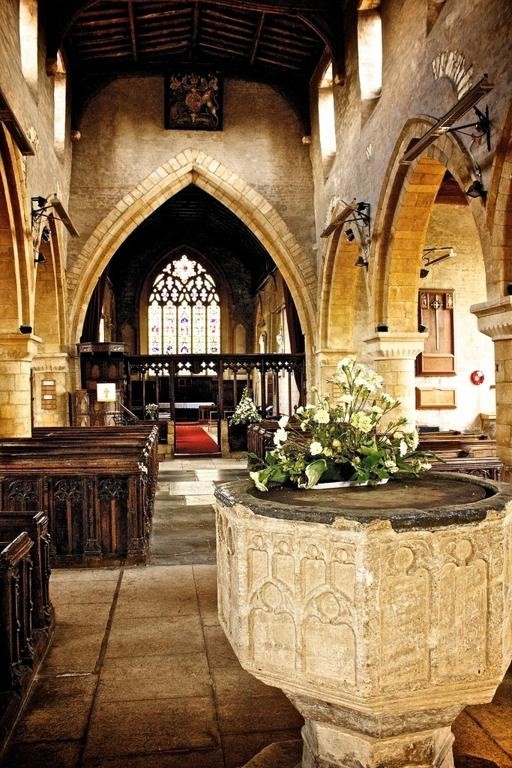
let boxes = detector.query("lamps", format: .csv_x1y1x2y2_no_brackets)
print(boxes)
464,179,487,205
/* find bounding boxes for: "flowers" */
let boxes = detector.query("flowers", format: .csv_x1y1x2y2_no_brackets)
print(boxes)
224,354,448,501
